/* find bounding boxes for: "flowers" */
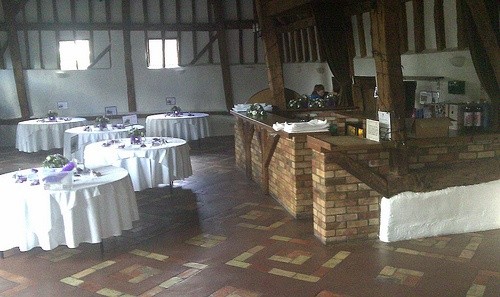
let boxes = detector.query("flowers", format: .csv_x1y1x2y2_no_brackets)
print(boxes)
45,109,58,118
248,103,265,117
94,116,110,127
42,154,71,169
170,106,183,114
128,127,146,142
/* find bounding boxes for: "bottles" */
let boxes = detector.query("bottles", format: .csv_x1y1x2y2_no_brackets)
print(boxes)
474,100,490,130
462,101,473,133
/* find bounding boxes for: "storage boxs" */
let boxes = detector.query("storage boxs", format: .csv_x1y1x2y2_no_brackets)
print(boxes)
403,115,456,138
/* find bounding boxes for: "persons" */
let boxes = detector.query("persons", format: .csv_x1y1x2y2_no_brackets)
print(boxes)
311,84,334,107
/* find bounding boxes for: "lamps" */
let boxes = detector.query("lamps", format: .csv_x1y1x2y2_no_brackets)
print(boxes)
250,21,264,38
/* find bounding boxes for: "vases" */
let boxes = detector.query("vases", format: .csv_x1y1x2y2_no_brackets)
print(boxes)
173,110,180,116
84,137,194,191
48,116,54,120
49,166,73,184
133,139,140,145
98,124,106,131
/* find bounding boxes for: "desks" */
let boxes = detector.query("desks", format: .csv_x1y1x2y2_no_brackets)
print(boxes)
0,164,140,258
145,112,211,149
64,126,143,164
16,118,86,152
